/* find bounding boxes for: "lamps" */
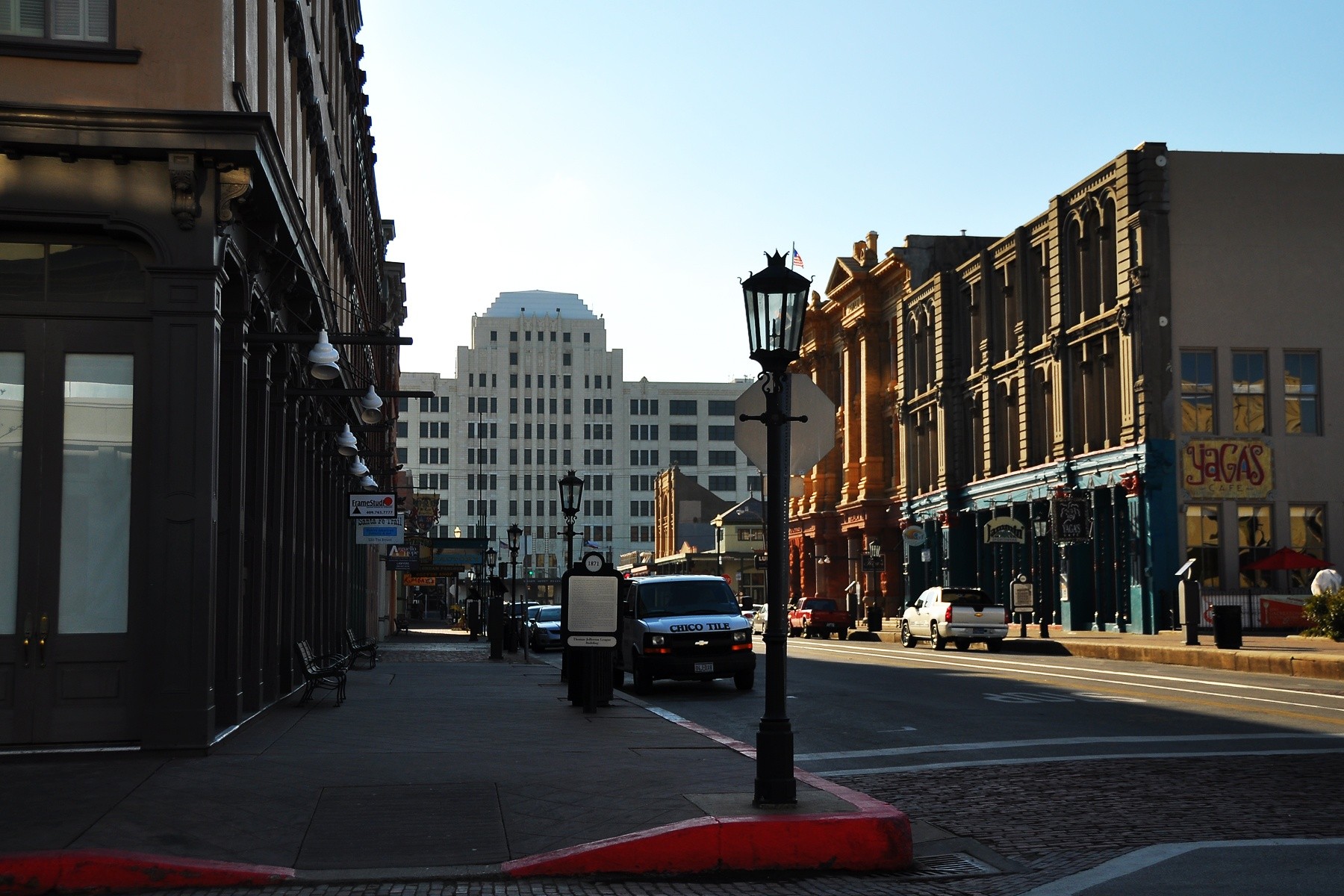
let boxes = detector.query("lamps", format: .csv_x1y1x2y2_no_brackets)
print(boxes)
308,324,383,491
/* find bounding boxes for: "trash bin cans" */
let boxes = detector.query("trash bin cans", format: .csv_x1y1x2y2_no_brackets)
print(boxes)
1208,604,1243,649
865,606,882,631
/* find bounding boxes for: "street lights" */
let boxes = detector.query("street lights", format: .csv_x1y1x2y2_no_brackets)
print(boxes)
486,547,498,642
714,513,722,577
557,467,585,571
736,249,817,810
506,522,524,654
454,525,461,624
868,537,882,609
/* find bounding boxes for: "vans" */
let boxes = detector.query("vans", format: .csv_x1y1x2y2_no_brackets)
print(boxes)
622,573,756,696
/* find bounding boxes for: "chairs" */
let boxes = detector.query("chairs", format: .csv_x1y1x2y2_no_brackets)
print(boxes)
393,617,408,635
294,627,378,708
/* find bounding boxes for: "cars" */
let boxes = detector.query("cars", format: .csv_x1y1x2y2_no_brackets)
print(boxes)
530,605,561,654
741,603,768,636
500,599,552,648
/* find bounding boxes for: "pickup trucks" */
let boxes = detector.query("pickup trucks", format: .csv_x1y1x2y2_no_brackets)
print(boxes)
900,585,1008,653
786,596,849,641
